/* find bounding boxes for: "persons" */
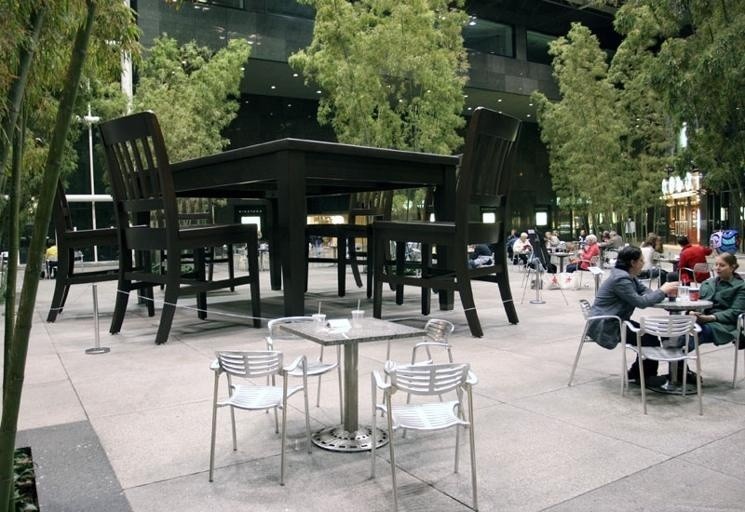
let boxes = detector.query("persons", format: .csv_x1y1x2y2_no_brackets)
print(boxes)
45,239,58,261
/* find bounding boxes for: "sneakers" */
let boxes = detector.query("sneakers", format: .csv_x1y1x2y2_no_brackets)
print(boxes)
627,369,667,387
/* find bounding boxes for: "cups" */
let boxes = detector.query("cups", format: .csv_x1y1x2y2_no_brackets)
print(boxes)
312,313,326,331
351,310,365,328
667,285,701,301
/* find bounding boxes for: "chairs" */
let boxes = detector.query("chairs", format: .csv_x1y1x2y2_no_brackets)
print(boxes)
208,349,312,486
265,315,344,433
731,313,745,388
513,252,526,268
572,256,599,290
622,314,703,414
33,135,155,324
97,110,262,345
160,193,235,293
366,153,459,305
382,318,469,439
373,106,523,338
568,298,629,390
369,360,479,512
305,189,397,298
679,262,716,287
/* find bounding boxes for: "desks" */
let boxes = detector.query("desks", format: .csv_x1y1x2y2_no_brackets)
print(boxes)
658,255,681,272
652,297,713,395
552,252,575,290
